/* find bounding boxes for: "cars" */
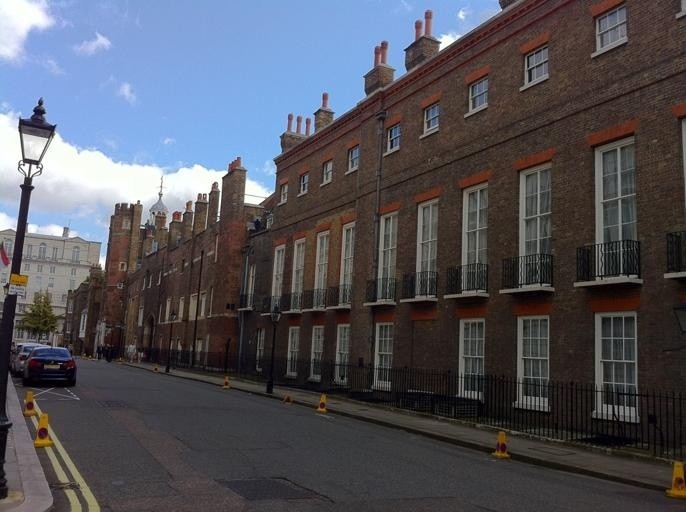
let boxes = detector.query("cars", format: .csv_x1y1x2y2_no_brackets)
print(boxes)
10,340,77,390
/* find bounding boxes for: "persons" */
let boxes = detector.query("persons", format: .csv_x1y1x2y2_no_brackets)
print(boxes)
94,340,144,366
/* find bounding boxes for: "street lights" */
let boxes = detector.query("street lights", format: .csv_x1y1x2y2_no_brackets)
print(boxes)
1,94,58,498
165,307,177,373
264,303,283,394
